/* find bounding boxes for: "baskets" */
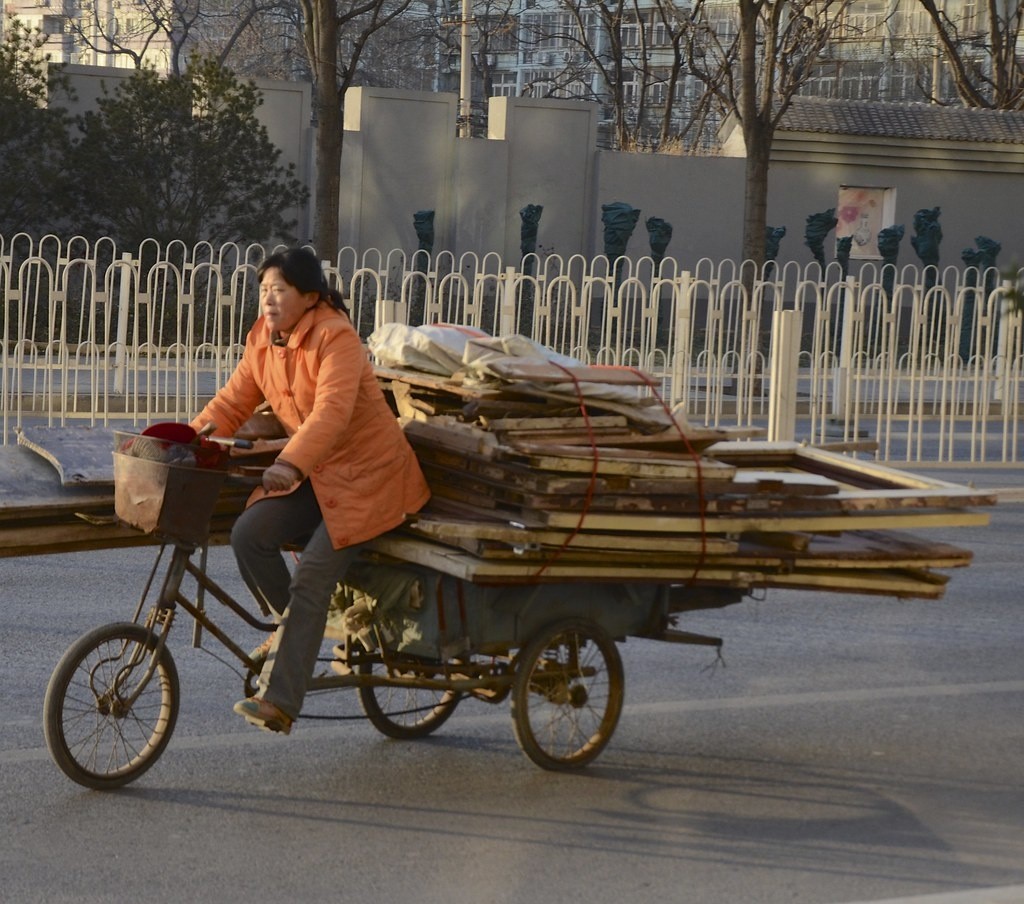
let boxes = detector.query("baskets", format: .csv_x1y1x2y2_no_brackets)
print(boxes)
113,429,231,548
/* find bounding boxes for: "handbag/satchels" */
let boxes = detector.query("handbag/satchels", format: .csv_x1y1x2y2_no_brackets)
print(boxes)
123,422,228,541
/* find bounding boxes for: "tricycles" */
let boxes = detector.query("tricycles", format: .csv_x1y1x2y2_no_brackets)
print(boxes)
41,427,674,790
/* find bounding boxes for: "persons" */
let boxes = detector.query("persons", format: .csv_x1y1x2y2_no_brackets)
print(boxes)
189,249,431,733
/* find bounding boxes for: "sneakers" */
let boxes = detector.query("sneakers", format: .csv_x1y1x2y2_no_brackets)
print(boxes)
233,696,292,735
242,629,276,669
